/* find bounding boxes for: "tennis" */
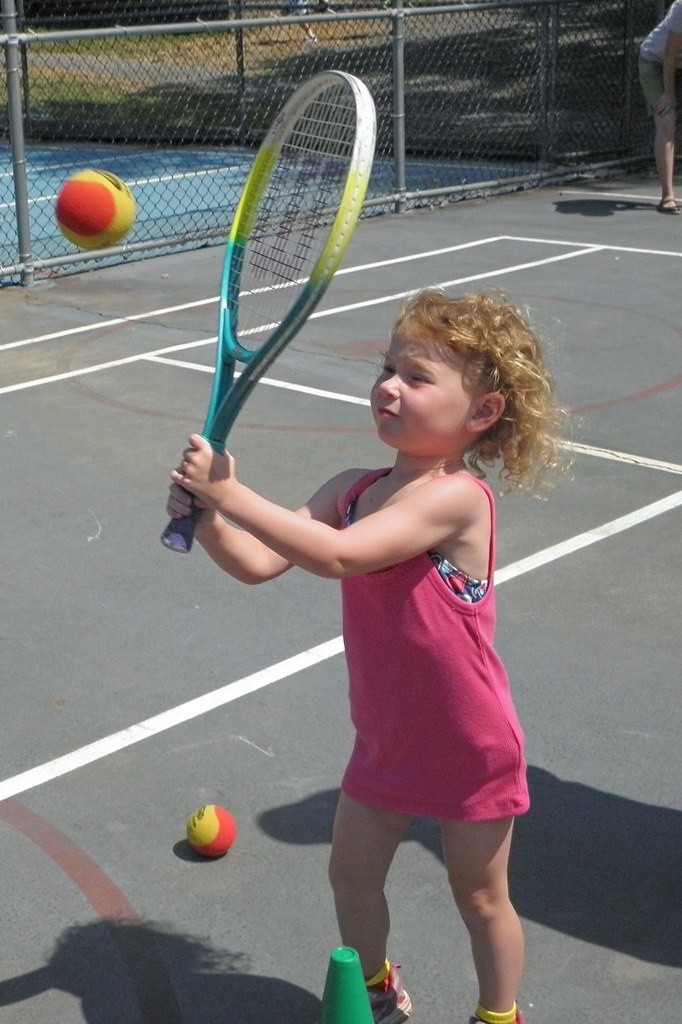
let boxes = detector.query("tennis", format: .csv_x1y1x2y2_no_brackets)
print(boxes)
186,804,237,858
54,168,137,251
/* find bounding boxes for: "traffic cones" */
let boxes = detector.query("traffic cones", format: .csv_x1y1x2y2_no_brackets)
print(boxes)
315,944,380,1024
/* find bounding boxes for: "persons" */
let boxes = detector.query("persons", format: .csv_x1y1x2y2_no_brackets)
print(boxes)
165,285,570,1024
282,0,337,44
638,0,682,214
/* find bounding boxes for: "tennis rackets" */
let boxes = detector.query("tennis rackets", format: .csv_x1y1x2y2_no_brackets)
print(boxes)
159,69,378,554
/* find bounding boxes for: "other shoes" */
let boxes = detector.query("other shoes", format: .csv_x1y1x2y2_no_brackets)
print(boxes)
365,959,412,1024
468,1001,525,1024
657,198,680,214
